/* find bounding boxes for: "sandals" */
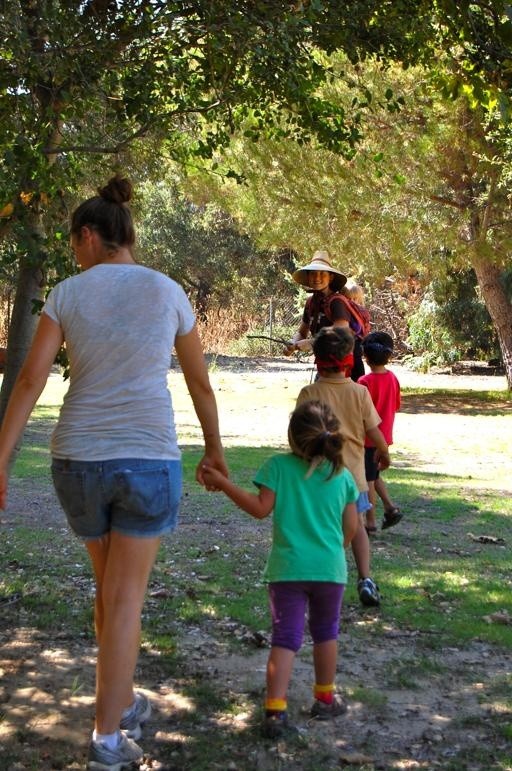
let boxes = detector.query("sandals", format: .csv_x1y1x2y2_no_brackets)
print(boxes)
379,504,403,532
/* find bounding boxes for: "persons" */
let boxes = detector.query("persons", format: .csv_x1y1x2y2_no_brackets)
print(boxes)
283,252,401,530
1,175,229,769
197,400,358,726
296,326,393,605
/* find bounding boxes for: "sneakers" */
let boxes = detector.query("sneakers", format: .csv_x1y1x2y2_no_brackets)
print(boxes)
119,689,152,741
260,706,290,738
357,577,382,607
309,692,346,722
85,730,145,771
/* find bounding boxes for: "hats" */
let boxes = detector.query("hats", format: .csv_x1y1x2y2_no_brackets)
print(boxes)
292,250,348,295
